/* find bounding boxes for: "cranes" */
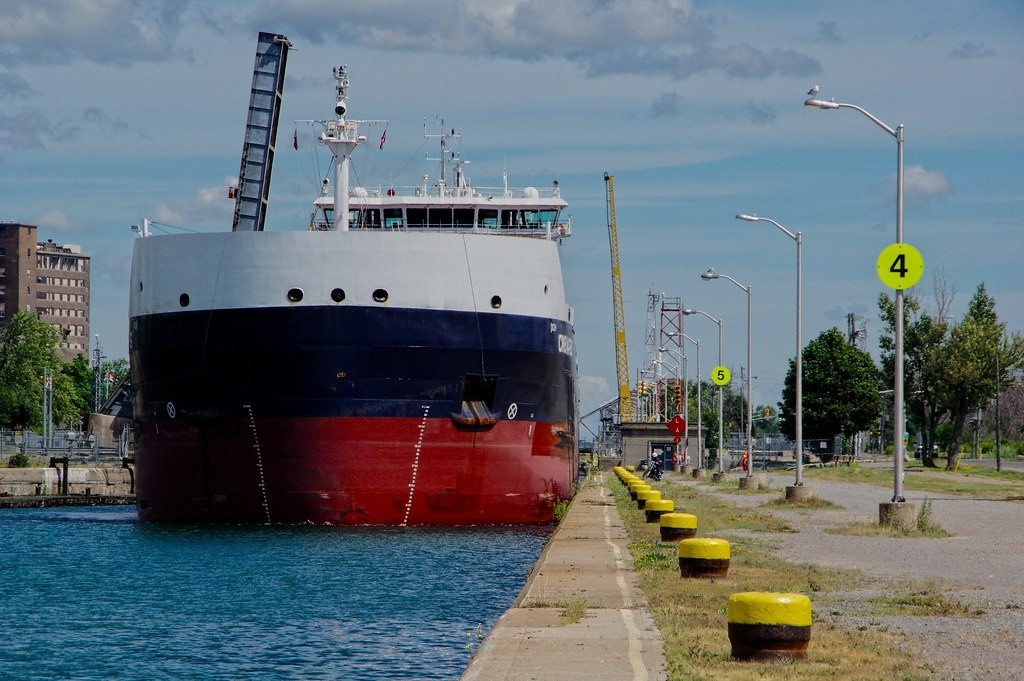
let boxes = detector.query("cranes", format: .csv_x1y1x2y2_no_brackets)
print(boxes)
604,172,635,426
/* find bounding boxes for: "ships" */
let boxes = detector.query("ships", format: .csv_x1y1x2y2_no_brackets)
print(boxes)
127,63,577,533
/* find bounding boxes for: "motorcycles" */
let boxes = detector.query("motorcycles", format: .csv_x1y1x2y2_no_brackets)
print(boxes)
643,456,664,482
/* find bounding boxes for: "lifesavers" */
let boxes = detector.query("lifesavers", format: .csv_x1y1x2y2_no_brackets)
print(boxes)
742,450,749,471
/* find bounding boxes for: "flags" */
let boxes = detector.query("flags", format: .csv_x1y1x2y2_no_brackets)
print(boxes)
106,372,115,382
294,129,298,150
380,130,386,149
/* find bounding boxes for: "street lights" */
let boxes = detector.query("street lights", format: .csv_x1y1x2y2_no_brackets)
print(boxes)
805,98,915,535
735,213,808,503
700,270,758,492
638,308,727,485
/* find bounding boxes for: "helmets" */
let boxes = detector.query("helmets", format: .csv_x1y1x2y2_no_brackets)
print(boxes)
652,453,658,457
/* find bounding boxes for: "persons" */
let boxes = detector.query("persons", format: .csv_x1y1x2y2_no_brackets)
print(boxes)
652,453,660,478
